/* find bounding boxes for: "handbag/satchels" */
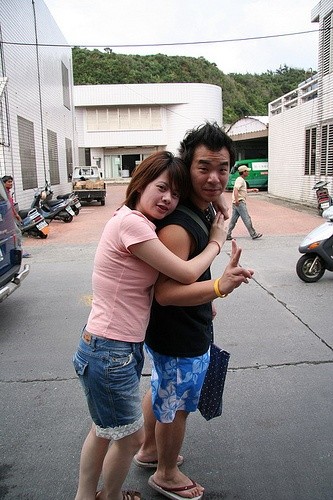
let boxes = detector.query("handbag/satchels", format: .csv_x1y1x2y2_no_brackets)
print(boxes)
196,322,230,421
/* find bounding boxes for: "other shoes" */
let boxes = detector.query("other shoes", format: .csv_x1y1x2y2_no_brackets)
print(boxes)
226,236,236,240
251,233,263,239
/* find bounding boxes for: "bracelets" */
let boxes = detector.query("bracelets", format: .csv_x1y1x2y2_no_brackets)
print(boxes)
214,278,229,298
251,189,254,192
207,241,221,255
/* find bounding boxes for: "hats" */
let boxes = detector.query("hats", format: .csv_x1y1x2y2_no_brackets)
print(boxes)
238,165,251,172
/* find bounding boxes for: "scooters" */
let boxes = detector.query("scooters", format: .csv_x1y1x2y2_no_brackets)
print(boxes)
28,180,81,223
296,205,333,283
311,180,333,216
12,192,49,239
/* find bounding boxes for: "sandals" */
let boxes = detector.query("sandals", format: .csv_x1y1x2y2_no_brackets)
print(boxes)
95,490,142,500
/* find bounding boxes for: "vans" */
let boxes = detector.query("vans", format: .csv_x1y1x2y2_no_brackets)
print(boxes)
226,159,268,191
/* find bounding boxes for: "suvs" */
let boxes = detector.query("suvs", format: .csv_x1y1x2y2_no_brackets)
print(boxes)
0,178,31,302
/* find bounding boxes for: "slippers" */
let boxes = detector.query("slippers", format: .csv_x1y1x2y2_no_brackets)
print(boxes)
148,474,203,500
132,454,184,470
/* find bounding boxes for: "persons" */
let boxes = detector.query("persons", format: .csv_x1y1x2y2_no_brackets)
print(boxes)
2,175,33,258
74,121,255,500
226,165,263,240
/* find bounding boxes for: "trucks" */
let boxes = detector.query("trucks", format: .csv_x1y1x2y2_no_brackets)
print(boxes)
70,166,106,206
